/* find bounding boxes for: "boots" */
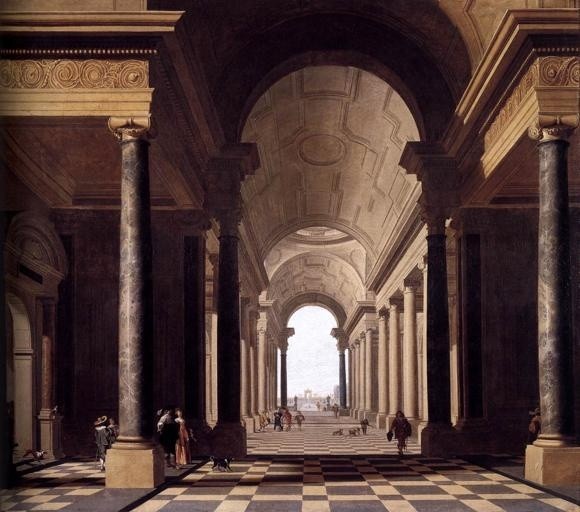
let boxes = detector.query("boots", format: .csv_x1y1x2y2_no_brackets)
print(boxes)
99,458,105,470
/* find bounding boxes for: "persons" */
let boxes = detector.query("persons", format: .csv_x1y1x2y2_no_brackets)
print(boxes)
156,408,177,467
527,408,542,444
173,408,194,465
107,417,119,445
390,410,409,454
360,417,370,435
258,405,305,432
94,416,112,471
332,403,339,418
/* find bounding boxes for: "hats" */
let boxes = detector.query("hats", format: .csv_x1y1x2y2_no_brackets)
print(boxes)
94,416,107,425
529,408,540,415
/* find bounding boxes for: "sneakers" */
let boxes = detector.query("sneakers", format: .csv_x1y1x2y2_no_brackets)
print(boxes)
167,464,179,470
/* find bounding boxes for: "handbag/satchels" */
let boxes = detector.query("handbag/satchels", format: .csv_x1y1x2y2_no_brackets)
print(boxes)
403,423,411,437
528,432,537,443
387,432,393,441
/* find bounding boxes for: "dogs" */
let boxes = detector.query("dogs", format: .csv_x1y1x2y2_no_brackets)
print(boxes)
332,428,344,436
349,428,360,436
210,455,235,472
22,449,47,464
